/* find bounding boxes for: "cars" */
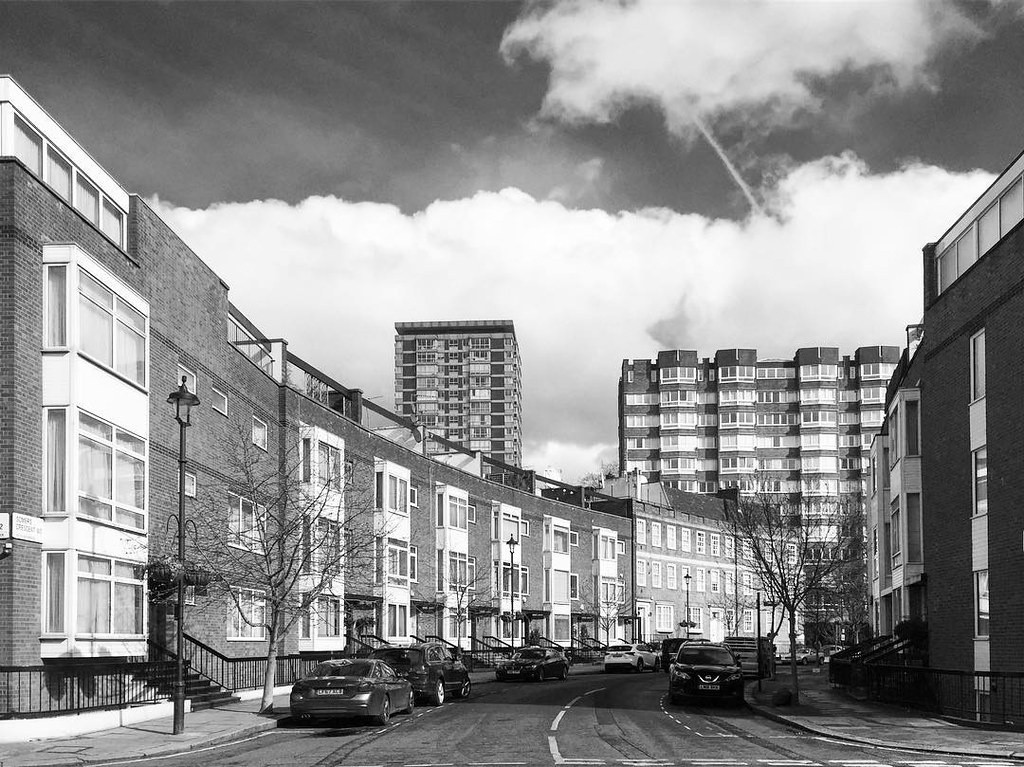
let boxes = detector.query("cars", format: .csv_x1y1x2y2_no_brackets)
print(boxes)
605,644,662,673
371,643,472,707
495,647,570,683
782,648,825,666
650,638,777,678
820,645,843,656
291,659,415,726
668,641,744,707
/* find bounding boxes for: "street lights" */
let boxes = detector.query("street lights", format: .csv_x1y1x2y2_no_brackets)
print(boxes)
683,574,692,638
166,375,201,734
506,534,518,648
